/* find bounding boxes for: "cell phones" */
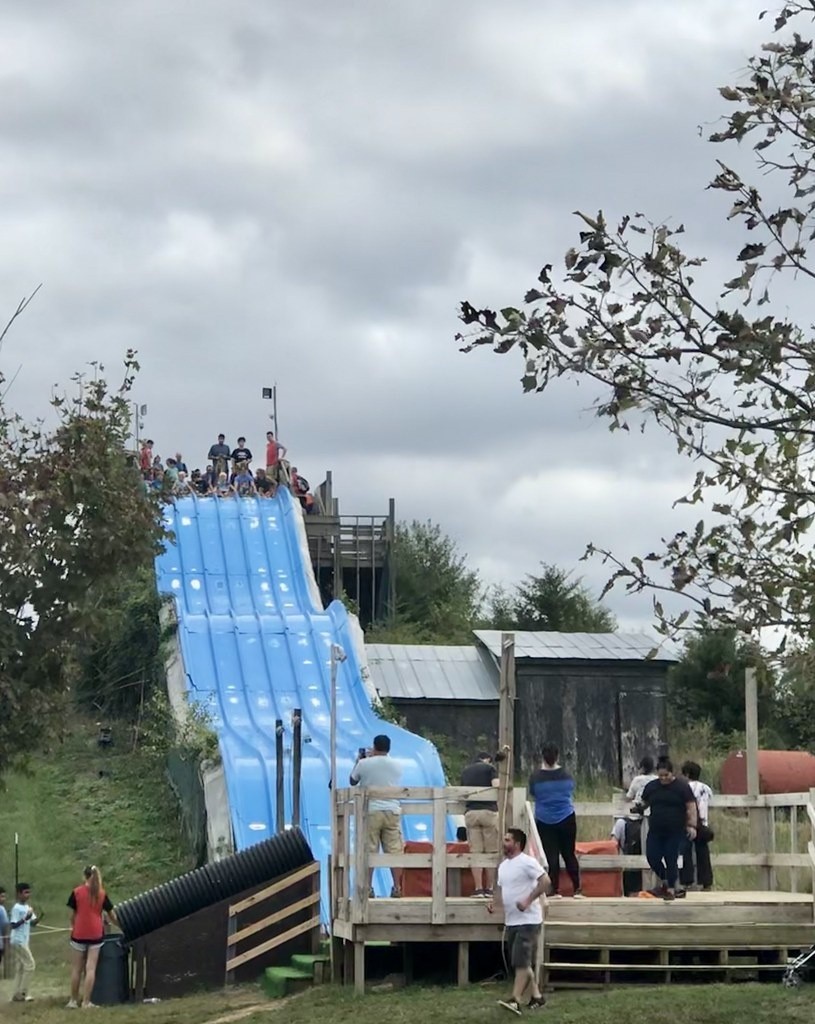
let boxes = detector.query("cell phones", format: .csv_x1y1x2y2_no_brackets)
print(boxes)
359,748,365,759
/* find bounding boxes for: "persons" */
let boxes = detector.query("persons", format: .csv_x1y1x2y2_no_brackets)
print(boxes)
452,740,712,901
0,883,44,1002
485,828,551,1017
66,864,117,1008
349,734,405,898
141,432,310,516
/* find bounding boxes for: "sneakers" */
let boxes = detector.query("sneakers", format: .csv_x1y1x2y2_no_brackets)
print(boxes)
663,890,675,900
548,890,562,898
65,999,79,1009
81,1000,100,1008
469,889,484,898
10,991,35,1002
573,888,589,897
485,889,495,897
528,995,546,1010
498,998,522,1016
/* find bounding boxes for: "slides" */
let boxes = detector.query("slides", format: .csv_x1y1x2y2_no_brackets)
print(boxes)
145,482,458,923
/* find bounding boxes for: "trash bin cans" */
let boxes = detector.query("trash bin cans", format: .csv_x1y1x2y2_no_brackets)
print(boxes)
85,934,129,1005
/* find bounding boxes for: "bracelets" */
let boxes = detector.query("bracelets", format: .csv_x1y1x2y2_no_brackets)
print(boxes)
687,824,695,829
23,918,28,923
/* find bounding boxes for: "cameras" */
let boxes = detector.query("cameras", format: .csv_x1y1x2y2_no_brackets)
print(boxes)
630,802,643,815
